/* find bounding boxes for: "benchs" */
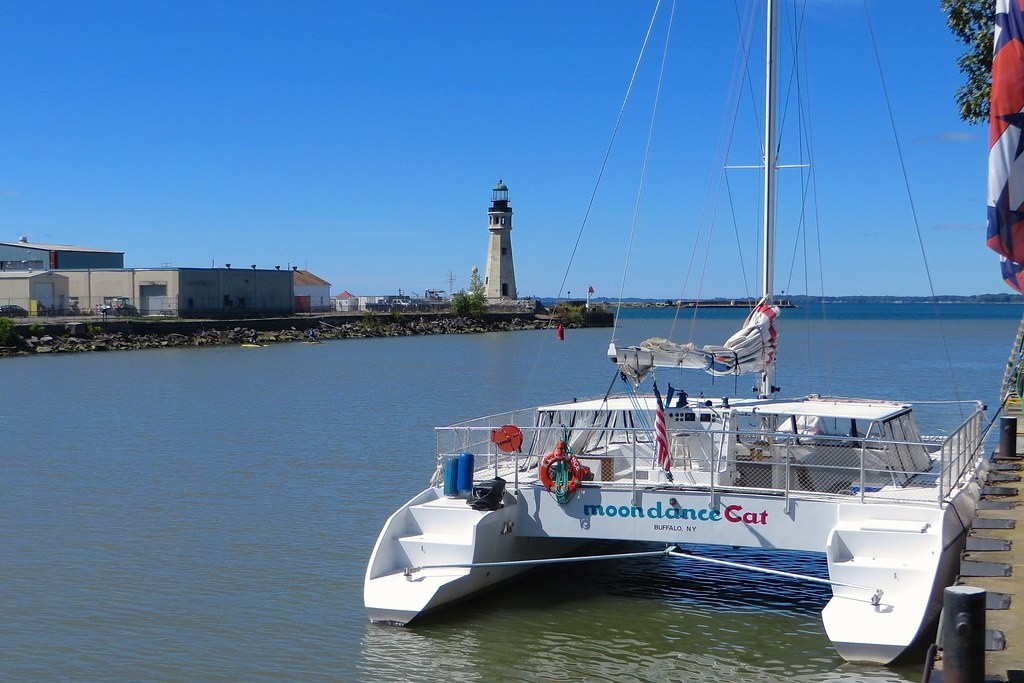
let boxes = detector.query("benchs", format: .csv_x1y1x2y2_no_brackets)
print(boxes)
157,309,176,316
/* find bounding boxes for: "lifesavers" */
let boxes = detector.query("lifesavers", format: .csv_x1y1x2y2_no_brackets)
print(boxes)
537,451,582,495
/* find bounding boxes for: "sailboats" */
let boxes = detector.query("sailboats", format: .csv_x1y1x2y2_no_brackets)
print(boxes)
358,0,991,666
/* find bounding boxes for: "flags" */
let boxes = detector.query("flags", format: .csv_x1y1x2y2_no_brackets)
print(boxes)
985,0,1024,296
654,398,671,472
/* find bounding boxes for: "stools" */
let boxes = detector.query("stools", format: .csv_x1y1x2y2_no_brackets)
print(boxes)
669,433,692,468
